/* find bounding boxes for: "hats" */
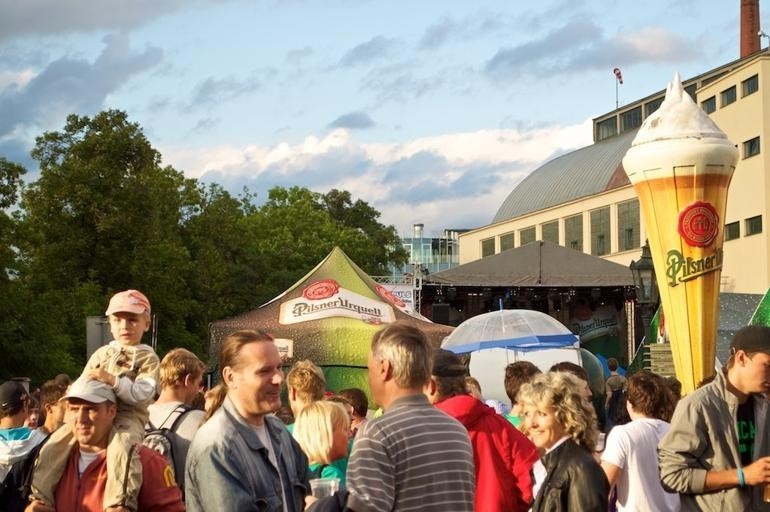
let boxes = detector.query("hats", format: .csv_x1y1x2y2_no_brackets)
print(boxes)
0,381,29,408
105,290,151,315
431,348,467,377
58,376,116,404
729,326,770,356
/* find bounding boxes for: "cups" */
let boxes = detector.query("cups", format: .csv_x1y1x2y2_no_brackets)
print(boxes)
310,476,340,500
594,433,606,453
763,482,769,502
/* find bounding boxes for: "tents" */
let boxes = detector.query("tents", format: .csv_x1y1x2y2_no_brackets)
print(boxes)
202,243,469,406
420,238,639,366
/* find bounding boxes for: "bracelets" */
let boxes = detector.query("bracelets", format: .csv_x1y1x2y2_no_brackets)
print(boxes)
737,467,744,487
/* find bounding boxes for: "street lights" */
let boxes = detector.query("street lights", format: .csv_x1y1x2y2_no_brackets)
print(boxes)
630,238,659,372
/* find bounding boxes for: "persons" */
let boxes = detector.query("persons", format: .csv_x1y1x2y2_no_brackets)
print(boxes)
141,349,207,501
23,396,39,428
304,323,475,511
501,360,544,429
0,380,48,512
604,358,715,426
465,377,481,395
423,349,547,512
40,380,66,437
184,329,309,512
655,324,770,512
515,371,611,512
293,401,353,492
24,373,185,512
327,387,368,432
549,361,592,402
28,289,160,512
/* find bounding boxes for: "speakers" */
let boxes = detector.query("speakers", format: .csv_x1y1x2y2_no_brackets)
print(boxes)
431,303,451,326
